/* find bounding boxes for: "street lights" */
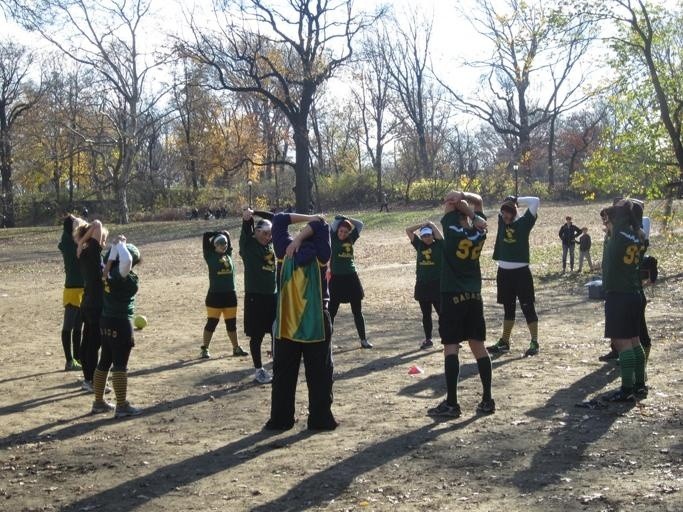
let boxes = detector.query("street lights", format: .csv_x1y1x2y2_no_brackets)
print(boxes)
246,180,252,210
512,164,519,206
1,192,7,228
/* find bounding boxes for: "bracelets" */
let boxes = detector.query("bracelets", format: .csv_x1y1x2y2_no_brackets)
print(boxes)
461,191,465,198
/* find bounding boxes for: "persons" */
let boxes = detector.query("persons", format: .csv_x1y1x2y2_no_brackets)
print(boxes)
405,220,462,348
559,216,582,274
238,207,278,383
575,227,593,272
265,211,339,430
427,189,495,417
486,196,539,357
380,192,389,212
328,213,373,348
200,230,249,358
599,197,651,403
57,213,144,418
189,207,227,222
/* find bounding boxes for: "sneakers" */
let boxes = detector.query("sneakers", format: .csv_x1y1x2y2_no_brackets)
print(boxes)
477,400,495,412
200,346,210,357
486,339,510,352
428,400,461,416
234,345,248,355
604,385,647,401
65,359,144,417
525,341,539,356
256,369,272,384
599,351,619,361
362,339,372,348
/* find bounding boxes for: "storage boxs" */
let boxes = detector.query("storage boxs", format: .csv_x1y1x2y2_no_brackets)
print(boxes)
588,284,603,300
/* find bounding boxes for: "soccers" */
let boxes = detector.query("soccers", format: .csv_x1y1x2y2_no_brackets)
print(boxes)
134,315,147,328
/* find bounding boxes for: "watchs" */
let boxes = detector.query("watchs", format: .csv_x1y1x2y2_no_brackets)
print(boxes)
472,214,478,222
